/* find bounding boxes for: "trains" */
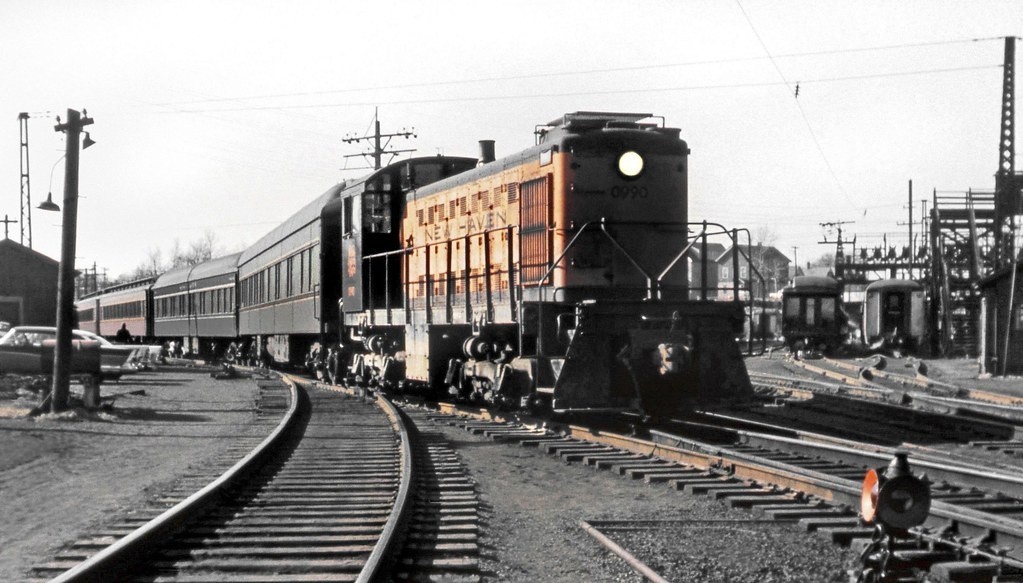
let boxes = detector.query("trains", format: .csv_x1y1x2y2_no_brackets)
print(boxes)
779,275,849,355
73,110,764,416
859,279,928,350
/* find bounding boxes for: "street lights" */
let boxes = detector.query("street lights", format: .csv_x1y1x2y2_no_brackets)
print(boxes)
34,128,97,414
790,246,798,276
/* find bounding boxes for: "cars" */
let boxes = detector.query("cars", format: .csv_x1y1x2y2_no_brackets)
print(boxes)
0,324,113,376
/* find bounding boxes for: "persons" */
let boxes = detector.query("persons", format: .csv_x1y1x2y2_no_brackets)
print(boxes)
114,322,132,344
